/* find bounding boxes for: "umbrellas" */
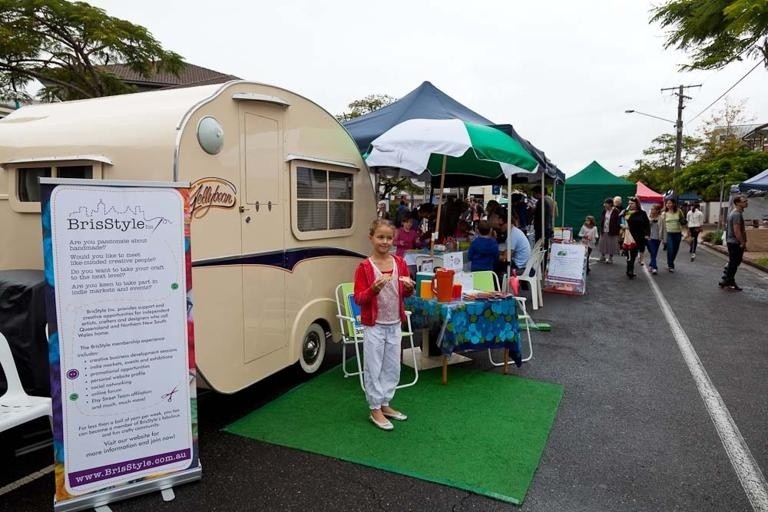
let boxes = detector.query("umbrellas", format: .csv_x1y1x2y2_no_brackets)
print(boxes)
362,119,540,256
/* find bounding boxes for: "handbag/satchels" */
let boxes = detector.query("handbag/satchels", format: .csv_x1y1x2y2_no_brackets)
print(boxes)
678,211,687,226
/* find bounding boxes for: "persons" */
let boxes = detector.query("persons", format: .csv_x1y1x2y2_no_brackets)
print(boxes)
719,196,748,291
376,185,559,291
354,217,416,430
578,196,705,280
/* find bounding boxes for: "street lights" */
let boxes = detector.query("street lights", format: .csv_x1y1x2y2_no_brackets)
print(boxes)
625,107,681,183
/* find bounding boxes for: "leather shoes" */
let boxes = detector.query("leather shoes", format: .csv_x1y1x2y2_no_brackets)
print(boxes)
382,410,408,422
369,414,394,432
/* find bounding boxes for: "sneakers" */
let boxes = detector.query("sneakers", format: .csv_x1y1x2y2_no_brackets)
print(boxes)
726,285,741,290
596,256,606,264
605,260,613,264
690,253,695,259
648,266,658,272
668,267,673,272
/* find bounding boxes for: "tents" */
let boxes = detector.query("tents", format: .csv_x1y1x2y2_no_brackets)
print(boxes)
555,161,637,244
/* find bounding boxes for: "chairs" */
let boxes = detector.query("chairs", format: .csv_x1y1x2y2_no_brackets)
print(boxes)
502,250,547,309
472,271,533,367
532,238,544,307
0,332,55,495
335,282,419,392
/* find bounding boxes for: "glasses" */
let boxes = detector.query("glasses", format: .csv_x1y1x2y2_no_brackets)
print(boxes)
498,222,504,226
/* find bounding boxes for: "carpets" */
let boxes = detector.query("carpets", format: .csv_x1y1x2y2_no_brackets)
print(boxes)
220,351,564,506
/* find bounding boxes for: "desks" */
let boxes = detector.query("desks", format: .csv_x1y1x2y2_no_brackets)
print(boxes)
405,249,473,273
403,289,522,371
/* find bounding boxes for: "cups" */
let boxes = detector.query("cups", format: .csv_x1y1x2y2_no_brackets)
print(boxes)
454,284,462,301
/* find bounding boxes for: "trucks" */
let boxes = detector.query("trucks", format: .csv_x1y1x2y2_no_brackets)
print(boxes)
1,82,378,505
